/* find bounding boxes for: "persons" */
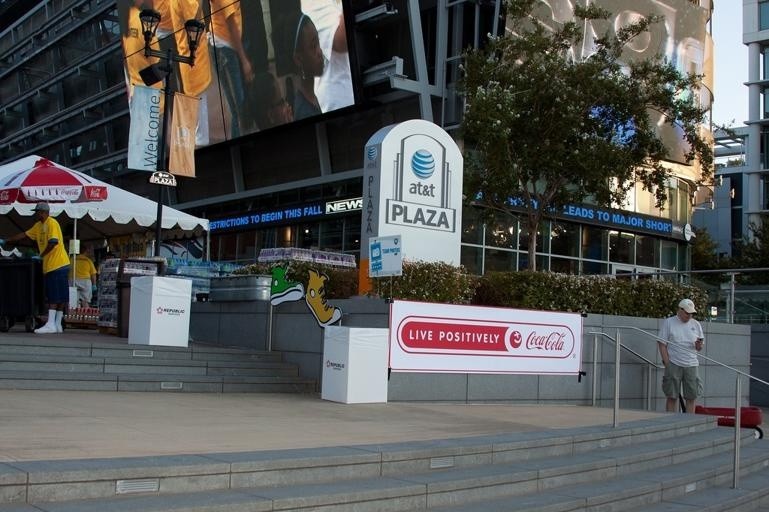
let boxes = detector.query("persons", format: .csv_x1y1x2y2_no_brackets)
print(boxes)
68,245,97,308
1,202,71,334
658,298,706,413
117,0,347,149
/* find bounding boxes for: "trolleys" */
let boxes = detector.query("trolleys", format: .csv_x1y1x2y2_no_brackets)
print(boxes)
672,381,764,442
0,256,66,333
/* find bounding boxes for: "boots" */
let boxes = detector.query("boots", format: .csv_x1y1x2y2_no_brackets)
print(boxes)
270,268,303,305
306,269,341,328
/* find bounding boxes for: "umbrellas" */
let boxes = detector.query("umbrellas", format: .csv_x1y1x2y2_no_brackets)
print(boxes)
0,158,108,205
0,154,210,286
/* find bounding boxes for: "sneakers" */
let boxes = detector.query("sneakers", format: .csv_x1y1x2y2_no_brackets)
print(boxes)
34,324,63,334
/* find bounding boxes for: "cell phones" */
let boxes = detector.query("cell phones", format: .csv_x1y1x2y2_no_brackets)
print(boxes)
697,337,704,345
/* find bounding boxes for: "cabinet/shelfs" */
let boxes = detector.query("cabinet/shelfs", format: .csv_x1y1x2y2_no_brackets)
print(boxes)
96,258,165,338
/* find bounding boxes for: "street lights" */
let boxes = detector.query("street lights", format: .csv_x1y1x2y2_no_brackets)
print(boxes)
138,6,206,260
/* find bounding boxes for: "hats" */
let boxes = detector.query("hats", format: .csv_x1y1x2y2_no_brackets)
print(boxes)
30,202,50,211
679,299,697,314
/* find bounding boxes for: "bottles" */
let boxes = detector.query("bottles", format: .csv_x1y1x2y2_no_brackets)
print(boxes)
98,301,117,327
63,304,99,321
95,256,244,301
258,248,356,268
